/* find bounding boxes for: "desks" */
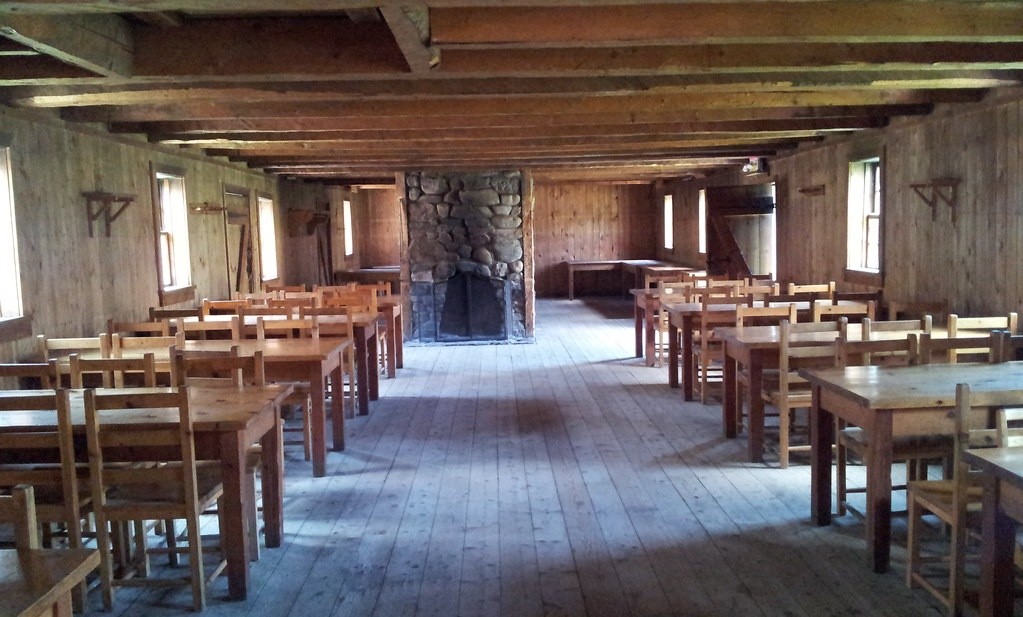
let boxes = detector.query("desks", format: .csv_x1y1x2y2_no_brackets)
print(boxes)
630,279,796,366
659,297,899,404
19,338,353,478
961,445,1023,617
713,323,1007,460
336,269,401,293
564,259,653,301
797,361,1023,575
0,384,294,600
134,312,383,416
0,547,101,617
210,294,404,379
622,260,705,288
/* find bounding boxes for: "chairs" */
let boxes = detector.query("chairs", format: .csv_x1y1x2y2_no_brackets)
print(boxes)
646,270,1023,617
0,279,405,617
